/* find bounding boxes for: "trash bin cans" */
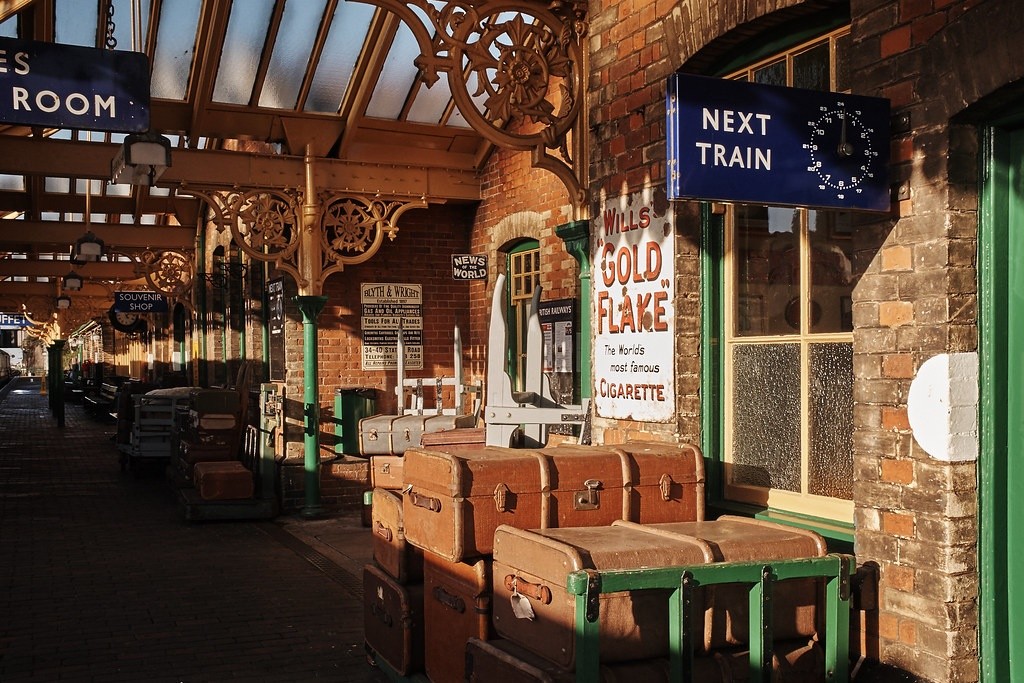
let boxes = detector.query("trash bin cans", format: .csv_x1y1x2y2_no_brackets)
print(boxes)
334,386,376,454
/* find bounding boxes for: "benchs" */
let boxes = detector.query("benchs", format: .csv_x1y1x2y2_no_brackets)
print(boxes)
85,382,119,420
116,393,190,459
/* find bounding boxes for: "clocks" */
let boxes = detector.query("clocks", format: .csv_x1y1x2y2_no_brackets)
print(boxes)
802,98,882,202
113,303,138,329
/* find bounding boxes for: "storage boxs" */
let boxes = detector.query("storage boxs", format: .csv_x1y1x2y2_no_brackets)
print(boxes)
370,454,405,489
422,549,492,683
62,358,282,526
363,560,424,678
490,514,828,673
420,427,486,450
358,413,475,456
401,439,706,564
370,486,425,582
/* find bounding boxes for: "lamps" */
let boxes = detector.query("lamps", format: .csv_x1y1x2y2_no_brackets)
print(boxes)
57,293,71,309
109,0,173,186
62,270,84,291
69,131,106,265
